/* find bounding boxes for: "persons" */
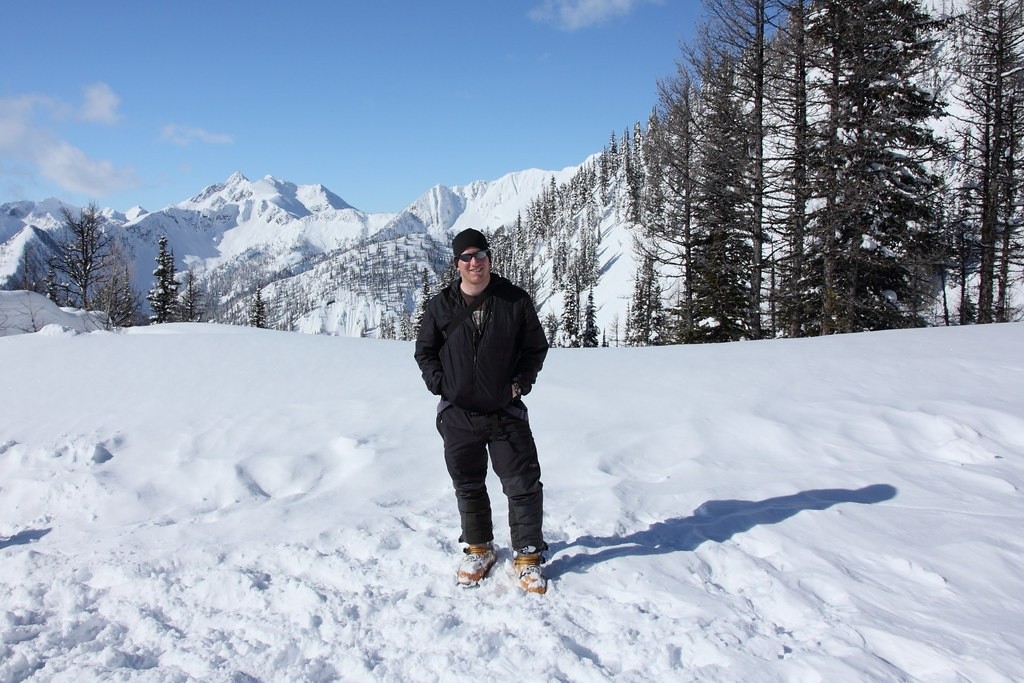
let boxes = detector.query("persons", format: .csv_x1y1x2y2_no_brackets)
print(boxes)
414,227,549,594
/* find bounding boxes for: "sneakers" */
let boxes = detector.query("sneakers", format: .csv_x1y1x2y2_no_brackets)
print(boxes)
456,542,497,587
513,550,546,595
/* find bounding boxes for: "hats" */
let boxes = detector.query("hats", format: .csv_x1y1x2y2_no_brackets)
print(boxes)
452,228,489,257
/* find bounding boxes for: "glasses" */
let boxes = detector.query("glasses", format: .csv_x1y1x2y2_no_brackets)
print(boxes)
458,249,489,262
